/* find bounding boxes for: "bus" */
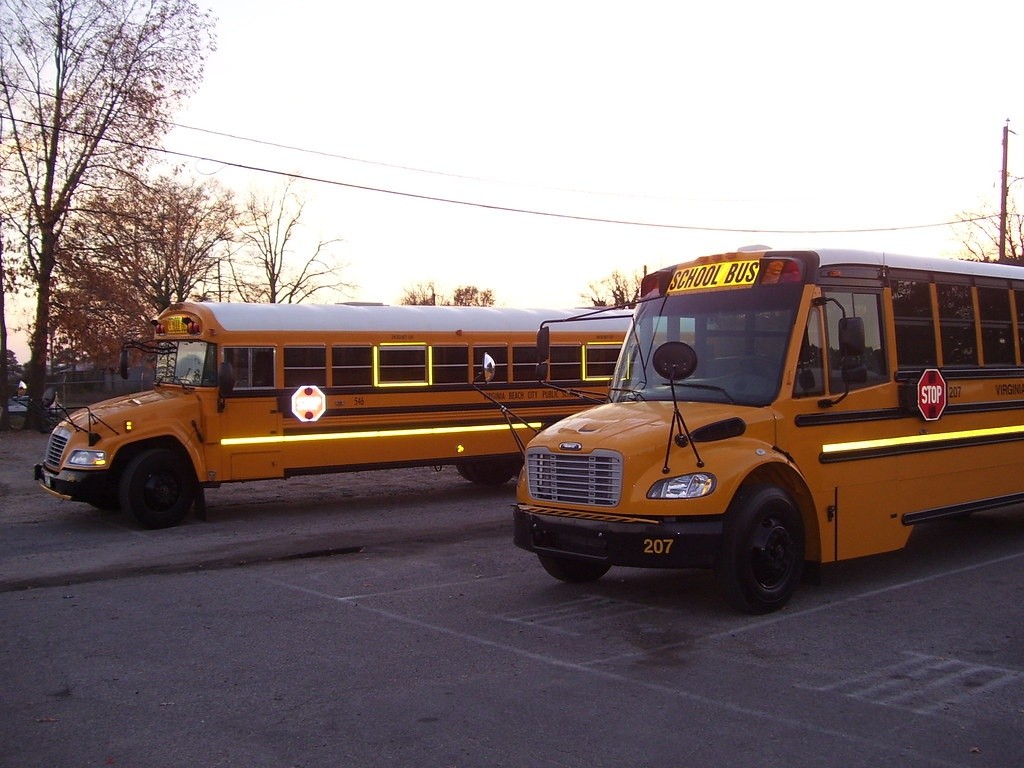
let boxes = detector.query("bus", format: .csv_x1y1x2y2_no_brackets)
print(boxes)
467,246,1024,617
10,303,716,529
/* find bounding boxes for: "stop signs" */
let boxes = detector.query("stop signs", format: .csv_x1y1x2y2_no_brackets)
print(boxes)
291,384,326,422
913,368,947,422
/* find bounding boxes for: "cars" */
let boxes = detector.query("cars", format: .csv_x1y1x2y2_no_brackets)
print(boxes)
8,396,62,414
